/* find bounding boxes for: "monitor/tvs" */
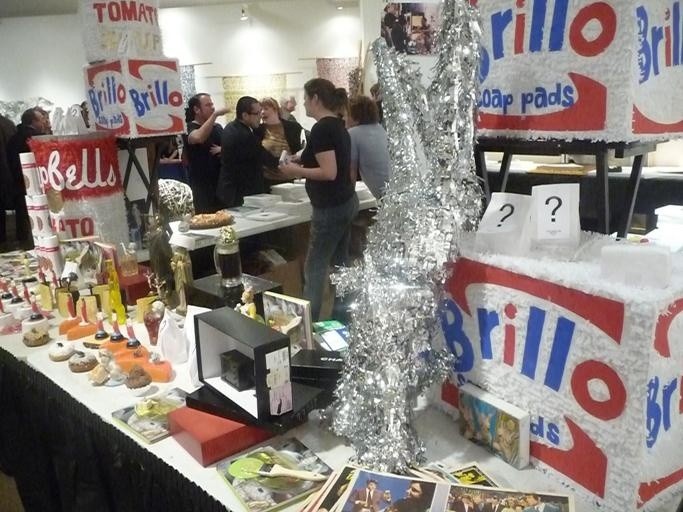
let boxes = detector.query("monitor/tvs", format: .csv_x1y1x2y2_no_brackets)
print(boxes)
294,464,574,512
458,379,530,469
109,386,193,443
214,436,338,512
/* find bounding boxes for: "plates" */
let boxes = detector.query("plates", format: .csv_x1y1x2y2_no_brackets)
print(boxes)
469,133,671,239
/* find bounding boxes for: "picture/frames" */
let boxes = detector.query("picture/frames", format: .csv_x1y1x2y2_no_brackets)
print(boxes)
0,245,563,512
165,191,381,252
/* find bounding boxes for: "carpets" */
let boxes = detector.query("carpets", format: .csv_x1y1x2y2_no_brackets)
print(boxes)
367,492,371,509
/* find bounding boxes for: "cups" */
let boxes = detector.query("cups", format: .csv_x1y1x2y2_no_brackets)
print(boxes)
248,109,263,117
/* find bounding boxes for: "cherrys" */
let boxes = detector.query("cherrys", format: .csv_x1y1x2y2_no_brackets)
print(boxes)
240,460,328,481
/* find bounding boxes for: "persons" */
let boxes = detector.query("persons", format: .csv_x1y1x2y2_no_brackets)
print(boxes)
341,94,396,203
0,105,49,253
277,77,357,320
333,87,349,132
155,93,315,273
79,99,90,129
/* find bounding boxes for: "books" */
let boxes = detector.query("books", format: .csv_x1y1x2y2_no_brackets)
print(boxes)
83,57,187,140
237,210,375,296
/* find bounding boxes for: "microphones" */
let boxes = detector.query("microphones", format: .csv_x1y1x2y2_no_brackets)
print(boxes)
107,132,179,247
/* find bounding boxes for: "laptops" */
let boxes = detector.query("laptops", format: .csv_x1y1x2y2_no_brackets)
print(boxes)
189,210,232,228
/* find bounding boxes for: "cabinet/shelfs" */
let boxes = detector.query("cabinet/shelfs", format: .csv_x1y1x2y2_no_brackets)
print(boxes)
17,151,65,280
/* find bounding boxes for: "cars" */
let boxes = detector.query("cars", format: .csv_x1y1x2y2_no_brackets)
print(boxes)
119,243,139,278
213,238,244,288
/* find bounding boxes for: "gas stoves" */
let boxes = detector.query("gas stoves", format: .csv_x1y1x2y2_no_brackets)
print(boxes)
0,255,171,397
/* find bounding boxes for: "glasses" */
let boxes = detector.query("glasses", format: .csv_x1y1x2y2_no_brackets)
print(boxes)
240,8,249,21
